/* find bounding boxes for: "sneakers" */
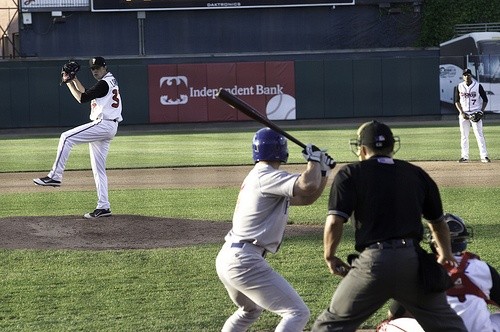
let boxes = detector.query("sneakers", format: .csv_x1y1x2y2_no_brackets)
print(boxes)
84,209,112,219
33,176,60,188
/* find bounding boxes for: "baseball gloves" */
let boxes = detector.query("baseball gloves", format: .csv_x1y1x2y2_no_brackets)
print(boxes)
59,61,80,86
468,110,484,122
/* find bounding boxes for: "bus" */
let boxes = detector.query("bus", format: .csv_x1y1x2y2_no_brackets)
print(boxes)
439,31,500,115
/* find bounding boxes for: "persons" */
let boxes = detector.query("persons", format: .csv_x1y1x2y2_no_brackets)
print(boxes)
214,125,331,332
309,122,470,332
452,71,493,164
32,55,121,220
376,210,500,332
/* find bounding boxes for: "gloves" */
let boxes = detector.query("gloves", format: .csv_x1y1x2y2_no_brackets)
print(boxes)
301,144,327,162
470,111,483,123
318,153,336,178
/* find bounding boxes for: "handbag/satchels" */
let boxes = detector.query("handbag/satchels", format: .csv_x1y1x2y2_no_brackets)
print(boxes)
414,244,454,292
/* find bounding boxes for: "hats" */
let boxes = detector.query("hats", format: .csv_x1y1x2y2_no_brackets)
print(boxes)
357,120,394,145
88,57,105,68
463,69,471,74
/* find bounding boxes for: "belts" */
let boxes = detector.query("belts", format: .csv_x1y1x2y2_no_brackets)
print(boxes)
368,237,417,250
231,243,244,248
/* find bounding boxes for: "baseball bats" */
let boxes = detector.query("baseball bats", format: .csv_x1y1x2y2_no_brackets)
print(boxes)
217,88,337,170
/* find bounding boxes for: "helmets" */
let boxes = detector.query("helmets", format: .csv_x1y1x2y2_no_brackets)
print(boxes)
430,214,468,256
252,127,288,164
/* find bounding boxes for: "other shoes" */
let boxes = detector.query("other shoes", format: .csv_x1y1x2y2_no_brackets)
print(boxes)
458,158,467,162
482,157,490,162
